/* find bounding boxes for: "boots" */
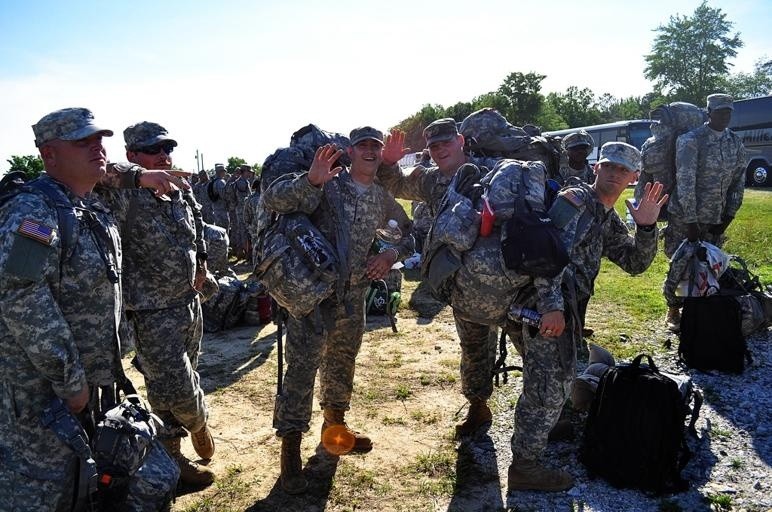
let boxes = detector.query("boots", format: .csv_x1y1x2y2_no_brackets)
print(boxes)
191,423,215,461
280,436,309,495
664,306,681,332
321,406,373,449
159,435,215,487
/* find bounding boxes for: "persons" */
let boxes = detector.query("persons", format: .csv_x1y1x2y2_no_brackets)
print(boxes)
96,121,214,477
0,168,27,205
503,141,669,492
0,109,124,511
662,94,748,333
262,125,414,495
377,116,526,437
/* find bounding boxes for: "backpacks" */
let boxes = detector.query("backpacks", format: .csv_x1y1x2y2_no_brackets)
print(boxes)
574,353,712,498
663,236,772,337
0,171,120,270
365,278,401,333
202,221,231,272
675,285,754,378
460,106,560,176
207,177,220,202
634,101,742,222
251,213,340,396
259,124,353,198
418,158,596,388
201,276,252,333
39,376,181,511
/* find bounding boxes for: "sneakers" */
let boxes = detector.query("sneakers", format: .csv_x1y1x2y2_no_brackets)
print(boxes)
508,462,575,492
456,405,492,436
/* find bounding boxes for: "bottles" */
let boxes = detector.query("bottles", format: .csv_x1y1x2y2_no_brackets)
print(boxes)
508,304,543,329
626,199,637,224
367,219,402,260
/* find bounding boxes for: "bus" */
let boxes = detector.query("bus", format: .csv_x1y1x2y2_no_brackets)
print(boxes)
397,151,434,169
727,94,772,188
540,119,655,189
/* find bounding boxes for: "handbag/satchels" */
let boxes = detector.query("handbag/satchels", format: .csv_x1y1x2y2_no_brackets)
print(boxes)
675,240,731,297
500,167,572,279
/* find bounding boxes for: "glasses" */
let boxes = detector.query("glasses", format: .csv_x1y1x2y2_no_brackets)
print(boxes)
129,142,174,155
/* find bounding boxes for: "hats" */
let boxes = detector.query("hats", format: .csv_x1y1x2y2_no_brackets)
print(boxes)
706,93,735,112
597,141,642,172
192,164,255,179
565,133,591,150
31,107,113,148
123,121,178,151
349,126,386,147
422,117,458,149
